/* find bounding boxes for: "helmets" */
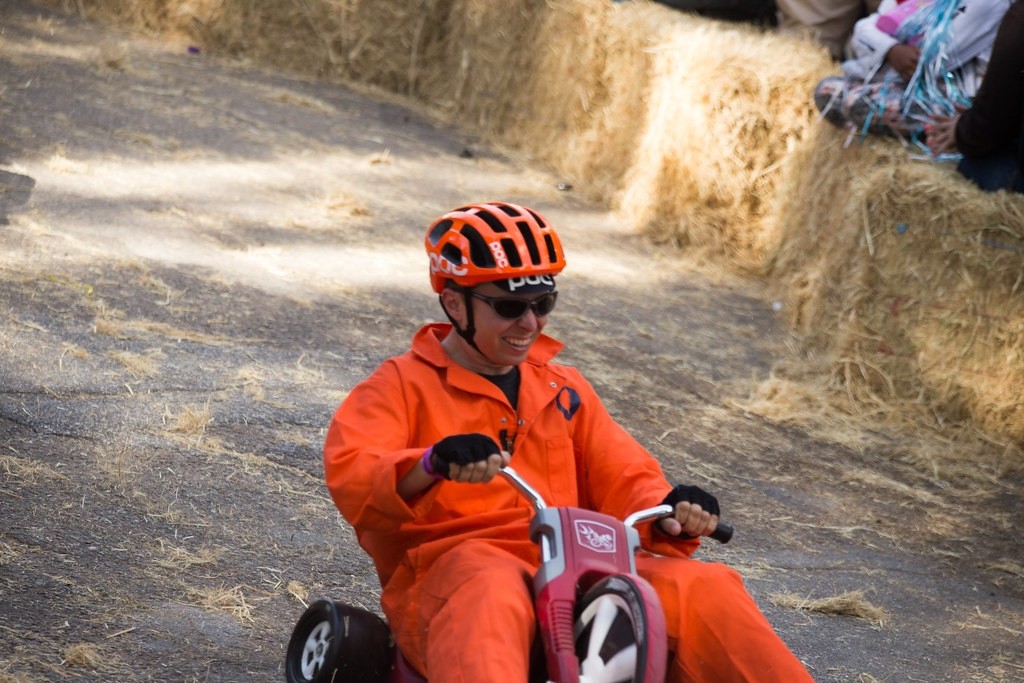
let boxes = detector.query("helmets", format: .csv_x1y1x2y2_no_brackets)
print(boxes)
424,200,566,296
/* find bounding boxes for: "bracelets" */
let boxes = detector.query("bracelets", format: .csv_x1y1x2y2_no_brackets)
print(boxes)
422,447,443,478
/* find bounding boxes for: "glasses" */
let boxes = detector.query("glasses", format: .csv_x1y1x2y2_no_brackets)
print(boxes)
464,289,559,320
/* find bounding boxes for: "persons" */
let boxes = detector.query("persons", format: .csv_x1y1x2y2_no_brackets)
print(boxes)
325,203,817,683
654,0,882,61
814,0,1015,143
925,0,1024,194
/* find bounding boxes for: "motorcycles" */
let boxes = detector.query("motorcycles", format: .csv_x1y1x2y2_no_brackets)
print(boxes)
284,462,734,683
